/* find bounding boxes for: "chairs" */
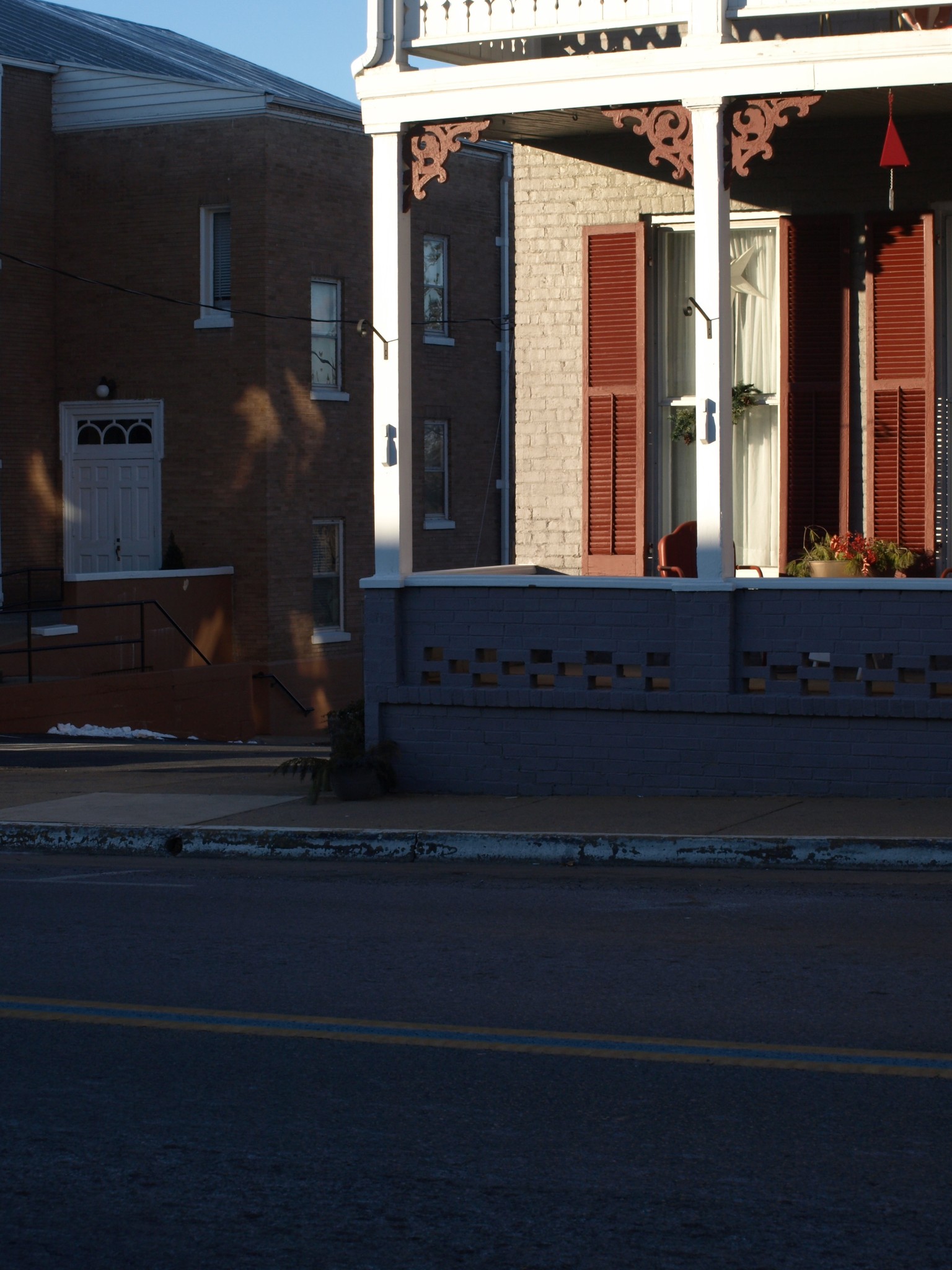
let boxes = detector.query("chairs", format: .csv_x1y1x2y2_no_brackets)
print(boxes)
655,522,764,578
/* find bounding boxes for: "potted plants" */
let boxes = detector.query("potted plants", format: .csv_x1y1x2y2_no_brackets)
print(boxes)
270,698,400,805
786,525,920,577
